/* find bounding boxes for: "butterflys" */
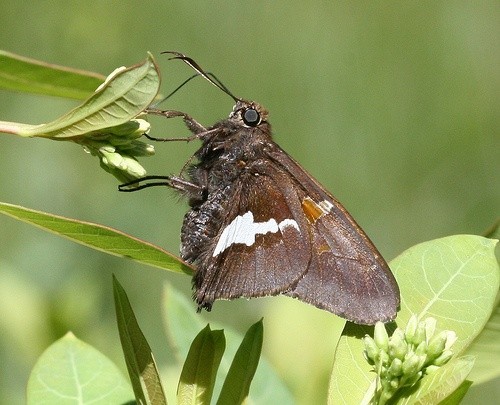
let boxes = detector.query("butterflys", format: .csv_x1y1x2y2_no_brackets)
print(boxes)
115,50,401,327
